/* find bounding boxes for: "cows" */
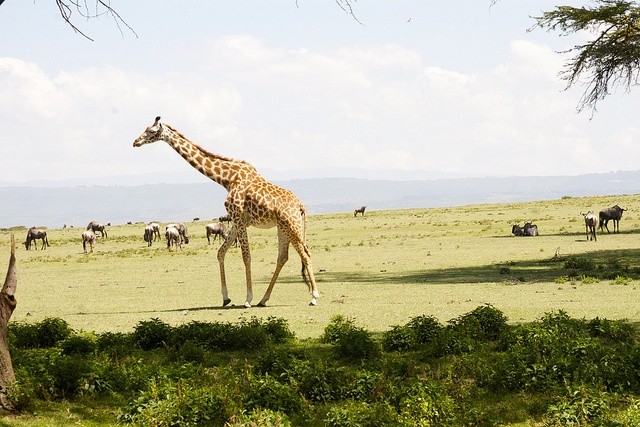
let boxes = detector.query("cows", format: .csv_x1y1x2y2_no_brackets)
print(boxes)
206,222,229,244
86,222,108,239
218,216,231,223
354,205,367,216
143,226,154,247
166,224,191,244
148,223,162,242
165,228,183,251
597,205,628,234
512,223,537,236
22,229,51,251
82,231,96,252
580,210,597,242
524,221,539,235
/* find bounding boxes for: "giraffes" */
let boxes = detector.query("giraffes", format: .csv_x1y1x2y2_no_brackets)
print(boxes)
133,116,319,309
